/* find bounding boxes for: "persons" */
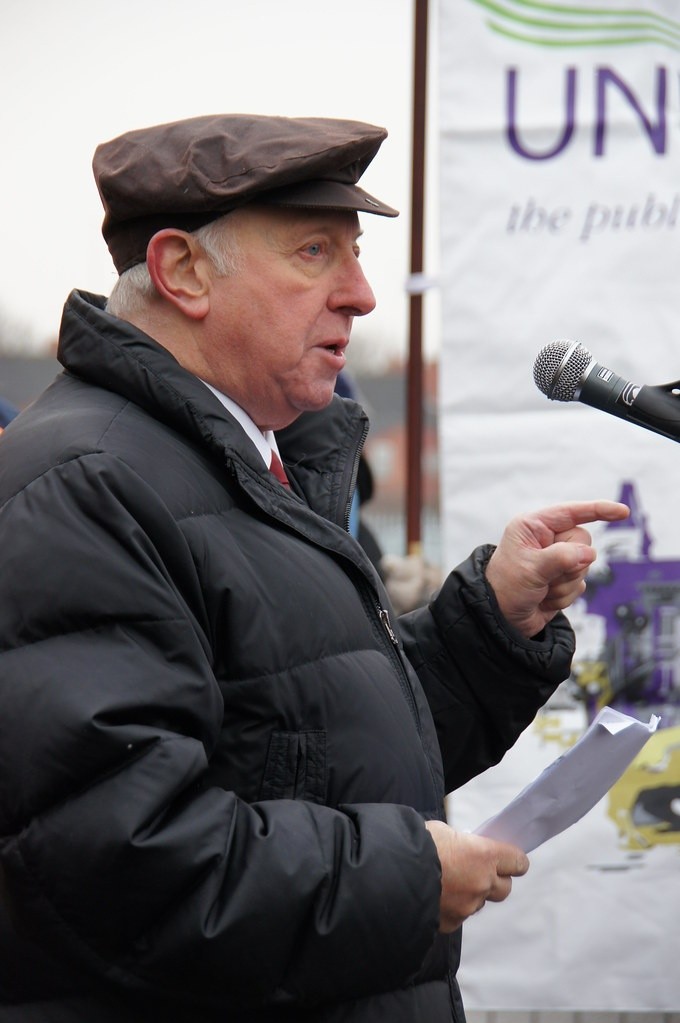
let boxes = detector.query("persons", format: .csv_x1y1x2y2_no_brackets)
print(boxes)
0,111,630,1023
0,359,431,626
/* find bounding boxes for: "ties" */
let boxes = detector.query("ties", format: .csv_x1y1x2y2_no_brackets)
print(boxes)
267,448,291,491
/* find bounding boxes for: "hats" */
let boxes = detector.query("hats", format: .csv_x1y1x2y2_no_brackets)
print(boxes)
92,114,399,276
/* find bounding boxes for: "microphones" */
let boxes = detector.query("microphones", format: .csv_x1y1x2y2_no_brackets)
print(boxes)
532,340,680,443
